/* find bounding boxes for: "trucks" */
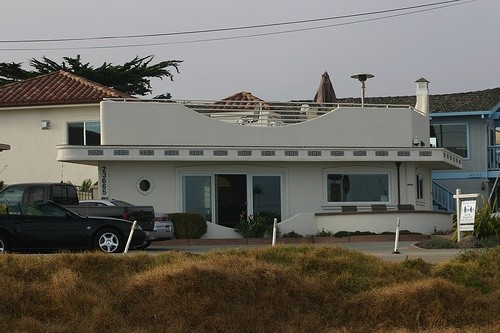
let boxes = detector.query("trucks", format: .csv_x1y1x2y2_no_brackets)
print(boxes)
0,181,156,233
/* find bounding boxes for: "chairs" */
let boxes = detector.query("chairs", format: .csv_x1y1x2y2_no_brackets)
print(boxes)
234,103,260,126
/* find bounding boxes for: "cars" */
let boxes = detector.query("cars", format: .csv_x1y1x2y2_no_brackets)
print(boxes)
1,198,147,253
77,199,174,248
